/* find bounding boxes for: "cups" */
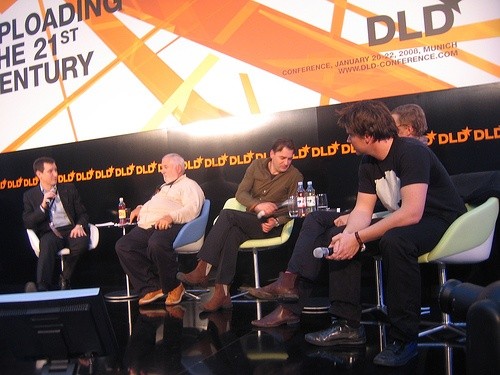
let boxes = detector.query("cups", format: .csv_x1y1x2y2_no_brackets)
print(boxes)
287,196,298,217
317,194,329,212
126,208,132,222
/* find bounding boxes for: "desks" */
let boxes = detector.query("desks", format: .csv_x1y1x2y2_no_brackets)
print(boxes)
95,221,140,299
287,208,351,311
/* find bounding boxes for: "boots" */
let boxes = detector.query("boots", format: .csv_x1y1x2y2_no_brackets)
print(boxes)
203,285,234,310
176,260,212,288
252,306,300,327
247,272,300,303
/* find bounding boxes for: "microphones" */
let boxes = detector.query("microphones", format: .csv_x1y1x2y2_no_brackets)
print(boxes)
47,187,56,207
314,247,334,258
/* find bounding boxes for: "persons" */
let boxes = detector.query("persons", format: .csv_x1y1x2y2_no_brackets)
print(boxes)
306,102,469,368
115,152,204,306
22,157,90,292
238,104,426,327
177,138,303,312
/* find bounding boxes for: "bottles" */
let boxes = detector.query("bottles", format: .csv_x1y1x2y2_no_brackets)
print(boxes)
118,197,126,224
306,180,317,214
296,181,307,218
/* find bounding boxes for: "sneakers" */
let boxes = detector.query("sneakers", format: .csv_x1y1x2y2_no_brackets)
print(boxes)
139,289,164,305
165,282,185,305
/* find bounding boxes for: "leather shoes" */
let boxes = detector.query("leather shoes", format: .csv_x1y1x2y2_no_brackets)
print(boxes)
59,272,73,290
373,340,417,366
26,282,38,292
306,316,366,344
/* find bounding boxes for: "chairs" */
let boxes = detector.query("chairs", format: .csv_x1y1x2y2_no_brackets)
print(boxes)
172,199,211,302
25,222,99,291
214,197,295,304
417,196,500,340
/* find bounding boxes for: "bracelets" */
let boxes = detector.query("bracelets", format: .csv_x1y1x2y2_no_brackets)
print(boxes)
275,219,279,227
355,232,362,246
81,225,83,227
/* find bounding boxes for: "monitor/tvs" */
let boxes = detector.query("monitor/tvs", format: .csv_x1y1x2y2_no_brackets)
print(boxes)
0,287,119,360
